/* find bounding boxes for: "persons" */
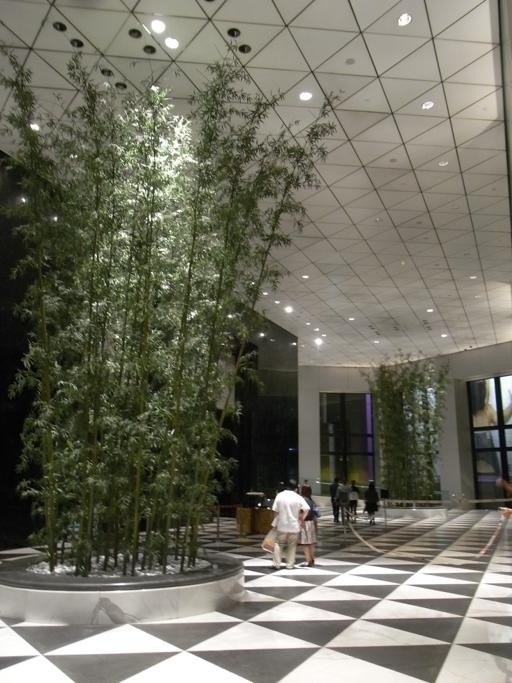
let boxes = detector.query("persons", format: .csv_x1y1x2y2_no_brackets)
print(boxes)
347,479,360,516
365,481,380,525
495,477,512,522
257,478,320,535
330,477,343,523
272,478,311,570
335,478,352,521
299,482,321,567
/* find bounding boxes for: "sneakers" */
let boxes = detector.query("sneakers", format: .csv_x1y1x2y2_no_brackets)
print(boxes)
273,561,315,570
